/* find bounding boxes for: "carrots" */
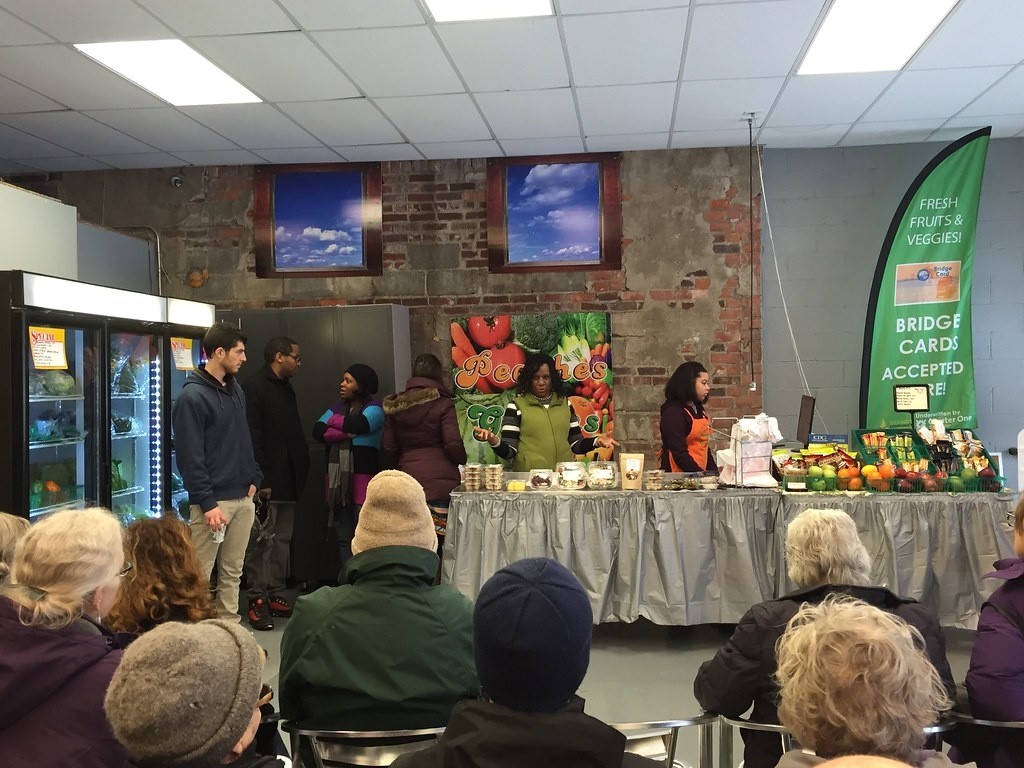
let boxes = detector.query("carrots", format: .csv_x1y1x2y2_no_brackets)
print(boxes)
590,384,613,426
590,342,609,358
451,323,492,393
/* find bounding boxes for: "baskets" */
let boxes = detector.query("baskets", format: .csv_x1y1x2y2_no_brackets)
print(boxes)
783,476,1006,494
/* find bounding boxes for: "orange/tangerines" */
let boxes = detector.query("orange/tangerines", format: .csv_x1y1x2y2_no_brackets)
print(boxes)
836,464,894,491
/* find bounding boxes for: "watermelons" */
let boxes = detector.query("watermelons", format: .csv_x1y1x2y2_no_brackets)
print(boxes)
453,390,519,466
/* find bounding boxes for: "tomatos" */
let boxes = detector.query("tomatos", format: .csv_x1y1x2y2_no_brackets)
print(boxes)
575,378,600,396
467,315,527,390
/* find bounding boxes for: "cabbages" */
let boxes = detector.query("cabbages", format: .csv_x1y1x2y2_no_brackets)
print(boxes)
40,369,76,396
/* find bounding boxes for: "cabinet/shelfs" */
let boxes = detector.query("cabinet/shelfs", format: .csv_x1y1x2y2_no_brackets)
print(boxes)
0,269,216,555
215,303,414,580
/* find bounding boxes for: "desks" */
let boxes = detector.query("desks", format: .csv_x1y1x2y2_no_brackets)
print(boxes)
443,468,1018,638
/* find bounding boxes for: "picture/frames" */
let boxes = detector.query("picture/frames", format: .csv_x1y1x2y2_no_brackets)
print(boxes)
486,152,623,274
254,160,385,278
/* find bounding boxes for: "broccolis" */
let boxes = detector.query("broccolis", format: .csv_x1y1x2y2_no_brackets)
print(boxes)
509,314,566,352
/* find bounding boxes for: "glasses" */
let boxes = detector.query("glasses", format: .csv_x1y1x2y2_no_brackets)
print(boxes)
115,561,133,577
255,684,274,709
1006,511,1023,527
288,354,304,363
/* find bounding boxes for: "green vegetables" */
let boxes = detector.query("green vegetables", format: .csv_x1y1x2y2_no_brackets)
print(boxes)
110,346,139,525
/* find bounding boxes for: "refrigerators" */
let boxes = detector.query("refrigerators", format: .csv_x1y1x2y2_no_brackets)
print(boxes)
0,269,216,527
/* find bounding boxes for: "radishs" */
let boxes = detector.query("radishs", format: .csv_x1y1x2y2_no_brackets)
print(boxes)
561,335,591,364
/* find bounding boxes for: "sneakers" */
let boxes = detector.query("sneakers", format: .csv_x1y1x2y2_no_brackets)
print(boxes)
270,597,294,617
249,597,274,631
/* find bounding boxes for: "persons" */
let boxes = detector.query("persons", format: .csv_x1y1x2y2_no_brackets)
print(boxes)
312,363,385,590
238,336,309,631
0,470,1024,768
172,323,269,658
472,354,620,473
383,353,468,559
654,361,719,476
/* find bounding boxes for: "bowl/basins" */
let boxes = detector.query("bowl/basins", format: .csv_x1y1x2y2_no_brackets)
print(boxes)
465,459,619,491
646,471,721,489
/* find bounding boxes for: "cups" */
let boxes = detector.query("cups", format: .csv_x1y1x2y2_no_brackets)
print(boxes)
210,523,226,543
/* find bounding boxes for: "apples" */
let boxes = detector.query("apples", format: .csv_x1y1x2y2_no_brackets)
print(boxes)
809,465,835,491
893,468,1001,492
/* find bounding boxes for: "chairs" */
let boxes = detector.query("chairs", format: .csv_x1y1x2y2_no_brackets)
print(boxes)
105,668,1024,768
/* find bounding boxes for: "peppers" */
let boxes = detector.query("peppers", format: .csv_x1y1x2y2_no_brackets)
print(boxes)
29,480,61,509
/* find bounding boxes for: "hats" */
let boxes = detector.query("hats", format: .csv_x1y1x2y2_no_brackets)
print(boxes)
414,353,442,376
103,619,265,768
346,364,378,394
351,470,438,556
473,557,593,713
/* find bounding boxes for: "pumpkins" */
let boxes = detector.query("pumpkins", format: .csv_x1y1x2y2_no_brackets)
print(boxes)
567,396,598,439
